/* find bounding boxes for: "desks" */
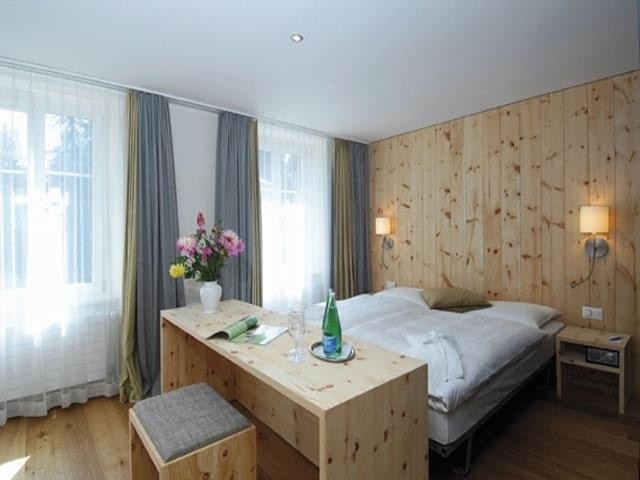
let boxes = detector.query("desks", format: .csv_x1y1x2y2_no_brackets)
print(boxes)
157,298,432,480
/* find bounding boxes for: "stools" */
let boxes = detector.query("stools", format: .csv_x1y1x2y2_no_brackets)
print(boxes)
125,381,260,480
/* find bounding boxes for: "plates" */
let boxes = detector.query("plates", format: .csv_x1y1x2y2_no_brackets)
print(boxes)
310,340,355,364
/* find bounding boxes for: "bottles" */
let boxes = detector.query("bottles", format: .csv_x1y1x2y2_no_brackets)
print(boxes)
322,289,345,359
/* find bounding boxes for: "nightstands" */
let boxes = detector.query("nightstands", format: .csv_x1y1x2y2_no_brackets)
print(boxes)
556,324,632,416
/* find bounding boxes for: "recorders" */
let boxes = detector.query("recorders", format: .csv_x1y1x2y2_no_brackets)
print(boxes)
585,345,620,367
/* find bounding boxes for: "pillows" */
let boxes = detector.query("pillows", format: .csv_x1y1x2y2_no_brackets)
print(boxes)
420,287,492,310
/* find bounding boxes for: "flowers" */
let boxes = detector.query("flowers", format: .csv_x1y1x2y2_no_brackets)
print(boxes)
166,209,249,285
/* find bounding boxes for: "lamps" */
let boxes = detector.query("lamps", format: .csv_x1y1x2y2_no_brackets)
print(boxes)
376,214,395,271
570,205,613,294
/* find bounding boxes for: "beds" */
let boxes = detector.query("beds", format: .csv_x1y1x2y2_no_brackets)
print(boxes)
299,283,566,474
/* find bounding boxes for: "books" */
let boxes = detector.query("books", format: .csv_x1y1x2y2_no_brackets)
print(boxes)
206,314,289,347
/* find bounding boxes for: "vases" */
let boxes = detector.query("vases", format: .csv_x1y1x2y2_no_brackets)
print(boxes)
199,279,223,316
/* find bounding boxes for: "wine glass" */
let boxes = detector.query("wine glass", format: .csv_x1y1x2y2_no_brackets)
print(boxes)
288,306,307,364
